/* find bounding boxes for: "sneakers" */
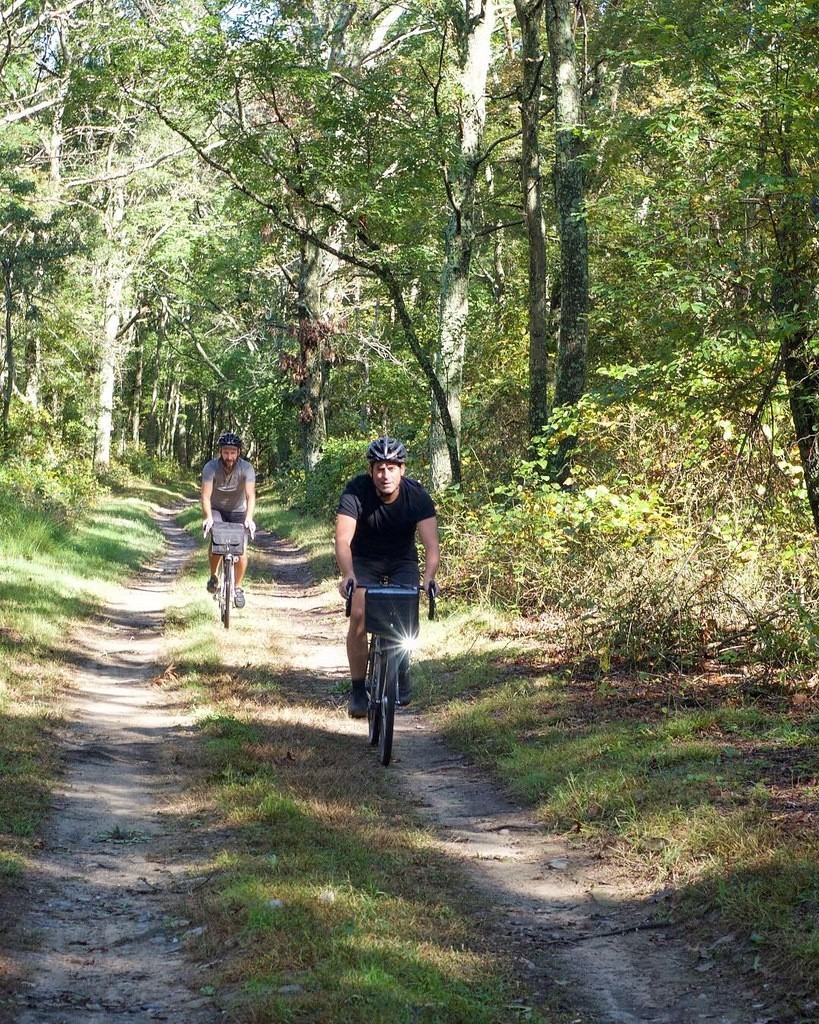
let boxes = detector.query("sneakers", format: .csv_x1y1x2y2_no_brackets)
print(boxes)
207,580,217,593
395,660,411,706
348,688,368,717
234,587,246,608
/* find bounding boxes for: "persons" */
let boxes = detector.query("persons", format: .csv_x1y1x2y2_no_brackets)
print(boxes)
201,433,256,608
335,436,441,717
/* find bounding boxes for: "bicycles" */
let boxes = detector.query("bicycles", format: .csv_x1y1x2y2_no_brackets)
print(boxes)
203,522,255,628
345,578,436,765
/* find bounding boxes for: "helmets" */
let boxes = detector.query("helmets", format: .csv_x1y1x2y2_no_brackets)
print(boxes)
366,437,407,463
217,433,242,446
